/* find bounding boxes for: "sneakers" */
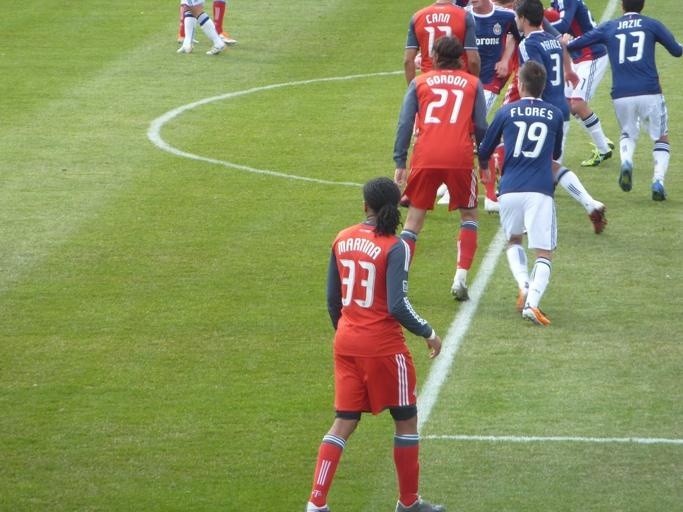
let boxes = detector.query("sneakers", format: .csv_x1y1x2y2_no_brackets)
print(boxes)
177,38,199,45
588,201,607,234
523,305,550,327
518,296,529,311
395,497,445,512
484,198,499,214
451,282,469,301
581,151,611,167
218,35,237,45
177,45,192,54
207,43,226,56
307,502,329,512
620,163,633,192
652,182,665,200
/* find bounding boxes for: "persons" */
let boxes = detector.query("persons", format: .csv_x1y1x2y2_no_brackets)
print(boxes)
405,1,480,207
463,0,524,189
176,0,236,55
563,1,683,200
540,1,612,167
515,1,606,235
393,38,488,300
305,178,446,511
478,61,563,326
